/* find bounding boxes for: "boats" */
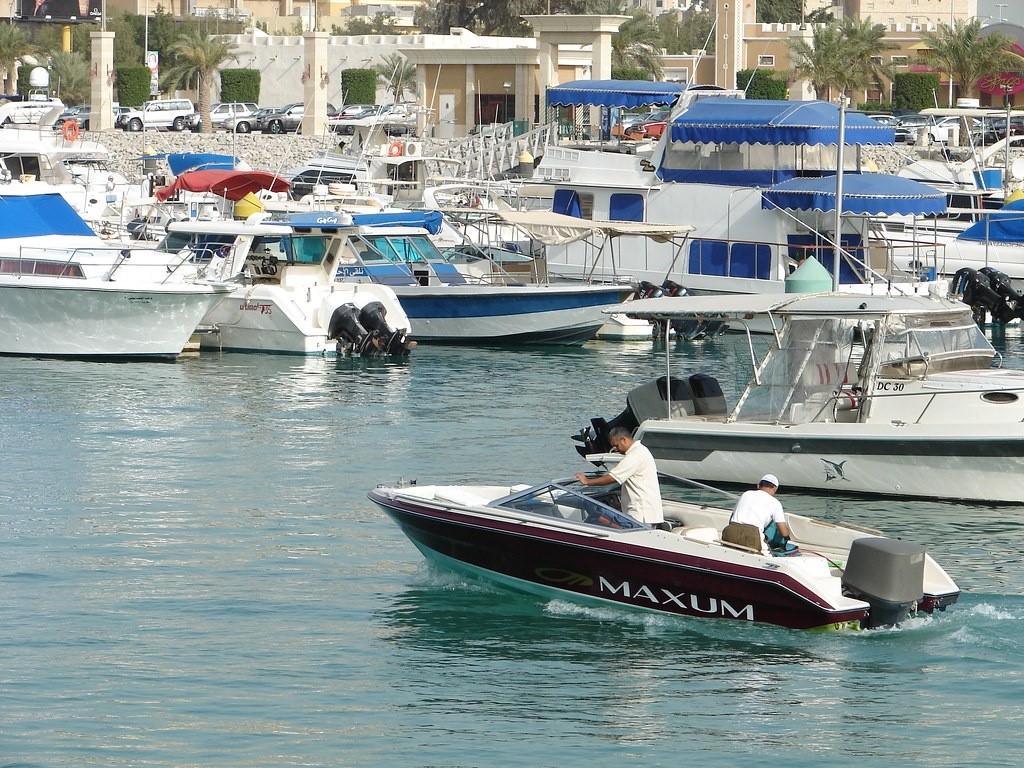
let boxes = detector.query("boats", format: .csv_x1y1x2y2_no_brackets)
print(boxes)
369,479,963,629
571,291,1024,504
0,181,238,363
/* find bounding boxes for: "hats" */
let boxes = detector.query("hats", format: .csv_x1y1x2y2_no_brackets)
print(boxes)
760,474,779,487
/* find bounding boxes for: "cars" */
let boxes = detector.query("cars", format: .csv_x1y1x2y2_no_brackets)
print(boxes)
326,101,416,136
220,108,284,133
976,118,1024,147
260,102,337,134
869,115,903,126
182,103,260,133
886,115,930,142
52,106,91,131
0,79,1024,363
613,112,671,141
905,115,990,147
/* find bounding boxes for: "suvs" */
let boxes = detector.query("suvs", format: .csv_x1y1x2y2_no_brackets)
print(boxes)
116,99,196,132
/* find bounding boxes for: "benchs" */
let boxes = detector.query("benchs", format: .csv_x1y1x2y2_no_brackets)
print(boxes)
801,362,859,410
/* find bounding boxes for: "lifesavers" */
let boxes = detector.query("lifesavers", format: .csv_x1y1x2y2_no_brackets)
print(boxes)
388,141,402,157
61,120,79,142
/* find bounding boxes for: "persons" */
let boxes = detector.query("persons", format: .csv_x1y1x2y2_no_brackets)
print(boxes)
575,426,664,532
726,471,790,552
